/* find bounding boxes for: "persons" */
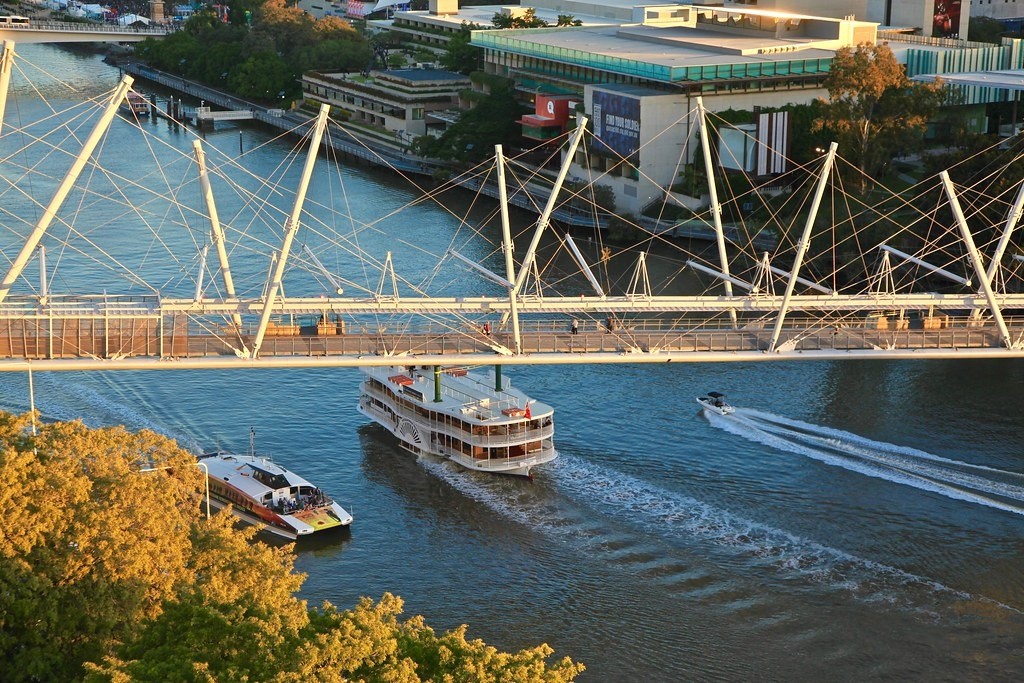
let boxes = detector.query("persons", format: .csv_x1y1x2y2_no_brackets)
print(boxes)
572,319,578,334
543,417,551,427
606,316,613,334
715,399,724,409
534,421,541,429
278,487,324,514
482,322,491,338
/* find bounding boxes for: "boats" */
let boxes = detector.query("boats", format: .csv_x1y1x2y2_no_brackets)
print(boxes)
355,350,559,481
696,391,735,416
150,426,353,540
119,90,150,116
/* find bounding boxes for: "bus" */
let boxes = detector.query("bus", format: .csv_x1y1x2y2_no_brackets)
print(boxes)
11,15,30,28
0,15,11,27
